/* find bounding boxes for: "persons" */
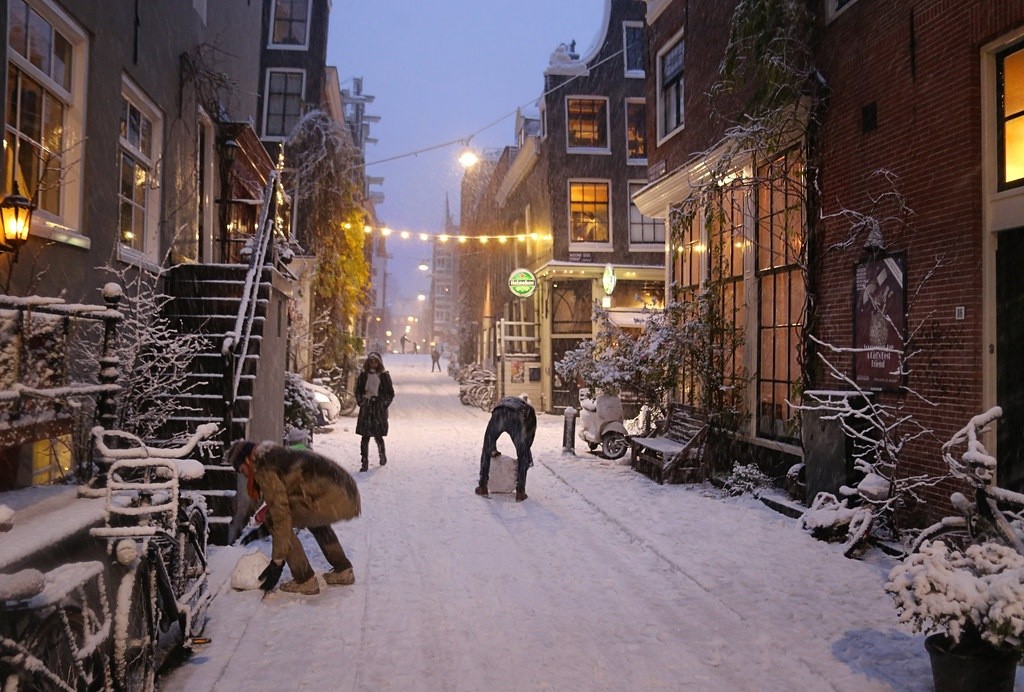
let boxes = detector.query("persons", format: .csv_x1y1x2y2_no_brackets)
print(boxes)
227,440,362,594
431,348,442,372
400,335,412,354
475,395,537,504
412,341,419,354
354,352,395,472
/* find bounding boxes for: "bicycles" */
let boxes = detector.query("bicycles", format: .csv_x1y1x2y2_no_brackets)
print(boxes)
447,350,496,413
800,460,895,556
318,368,357,416
911,406,1024,554
88,424,220,691
1,507,116,692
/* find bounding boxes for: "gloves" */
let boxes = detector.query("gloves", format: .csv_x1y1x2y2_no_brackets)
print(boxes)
239,523,270,546
258,559,283,592
492,451,501,458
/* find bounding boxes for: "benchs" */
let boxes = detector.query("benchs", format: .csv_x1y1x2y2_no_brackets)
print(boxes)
625,401,717,484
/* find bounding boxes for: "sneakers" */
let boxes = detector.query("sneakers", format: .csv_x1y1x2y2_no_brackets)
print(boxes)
321,567,355,585
280,577,320,596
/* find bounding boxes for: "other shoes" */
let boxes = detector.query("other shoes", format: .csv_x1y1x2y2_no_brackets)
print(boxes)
516,492,528,502
475,486,488,496
380,455,387,465
359,461,369,472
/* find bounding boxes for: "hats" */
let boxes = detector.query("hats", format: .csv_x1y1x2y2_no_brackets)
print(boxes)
226,440,255,472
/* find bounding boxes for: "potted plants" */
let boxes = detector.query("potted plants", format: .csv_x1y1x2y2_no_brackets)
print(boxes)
883,539,1024,692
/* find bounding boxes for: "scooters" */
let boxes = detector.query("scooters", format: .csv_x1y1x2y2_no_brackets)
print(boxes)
579,386,629,459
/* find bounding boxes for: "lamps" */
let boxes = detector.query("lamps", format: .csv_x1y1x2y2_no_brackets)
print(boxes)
0,180,38,264
457,136,479,169
